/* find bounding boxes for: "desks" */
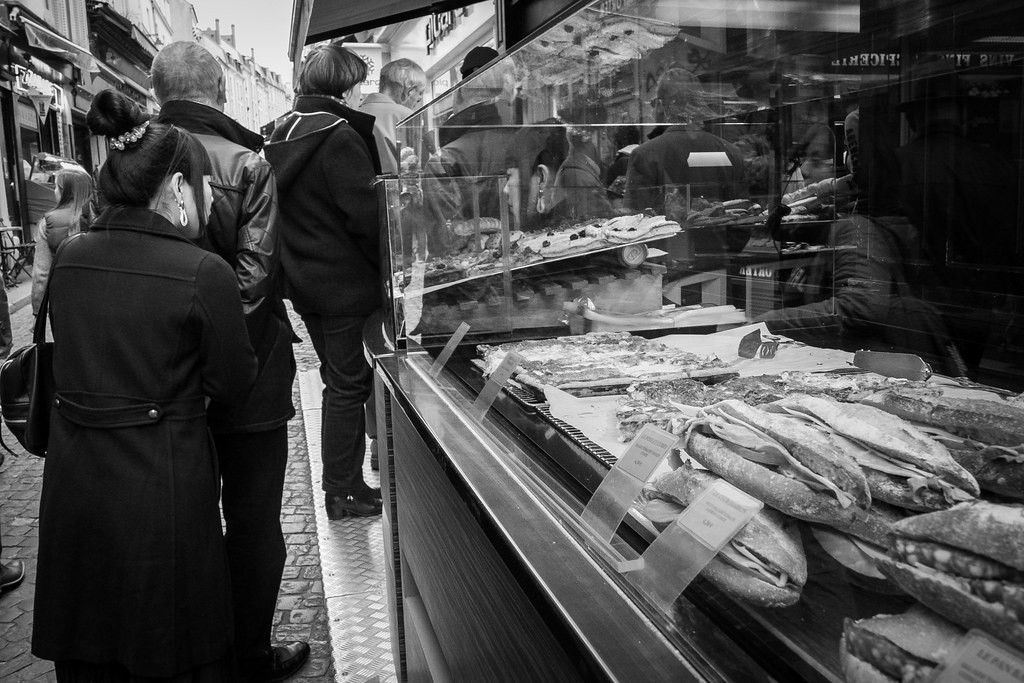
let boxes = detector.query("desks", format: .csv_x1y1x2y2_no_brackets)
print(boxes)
0,226,23,287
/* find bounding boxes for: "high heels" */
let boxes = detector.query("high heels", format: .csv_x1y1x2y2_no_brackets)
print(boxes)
325,487,383,520
359,481,382,498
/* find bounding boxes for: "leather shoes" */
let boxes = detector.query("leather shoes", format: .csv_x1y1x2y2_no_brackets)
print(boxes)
265,639,310,683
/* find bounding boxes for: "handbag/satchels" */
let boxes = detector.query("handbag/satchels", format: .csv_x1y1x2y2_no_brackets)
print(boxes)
0,231,84,457
812,221,965,382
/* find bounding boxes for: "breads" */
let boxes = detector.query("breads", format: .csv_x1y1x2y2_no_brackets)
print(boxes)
394,217,681,290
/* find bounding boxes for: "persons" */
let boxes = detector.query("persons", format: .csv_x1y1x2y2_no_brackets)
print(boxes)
0,41,502,683
420,94,996,374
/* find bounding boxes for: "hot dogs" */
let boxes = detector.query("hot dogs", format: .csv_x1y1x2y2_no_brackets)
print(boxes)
645,390,1023,683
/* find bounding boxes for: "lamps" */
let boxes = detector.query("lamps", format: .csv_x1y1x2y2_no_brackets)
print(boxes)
148,33,164,46
101,49,124,65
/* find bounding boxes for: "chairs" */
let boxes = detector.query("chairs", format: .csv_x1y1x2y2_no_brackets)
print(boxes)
0,218,37,289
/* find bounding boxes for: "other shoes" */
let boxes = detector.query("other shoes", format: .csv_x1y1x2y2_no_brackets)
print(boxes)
0,559,25,593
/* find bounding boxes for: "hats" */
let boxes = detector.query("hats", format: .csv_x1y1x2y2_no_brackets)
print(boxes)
460,48,502,73
557,94,606,131
